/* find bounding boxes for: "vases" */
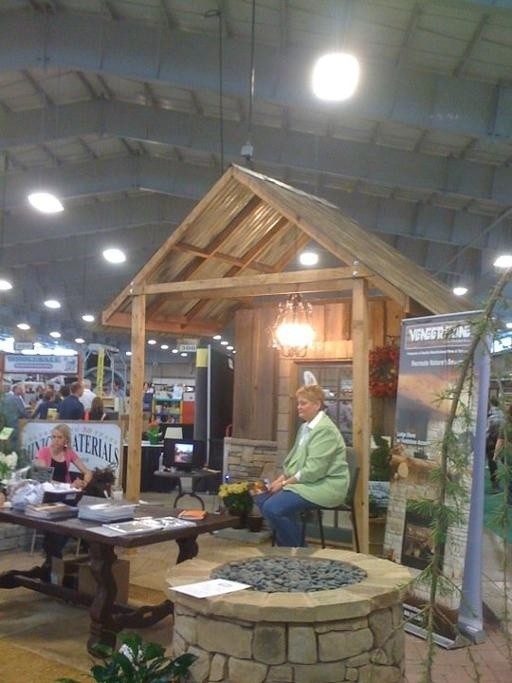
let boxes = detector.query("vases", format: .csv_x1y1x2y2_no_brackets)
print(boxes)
223,494,253,516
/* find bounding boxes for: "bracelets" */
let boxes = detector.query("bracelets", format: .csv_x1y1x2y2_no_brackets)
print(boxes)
282,480,287,487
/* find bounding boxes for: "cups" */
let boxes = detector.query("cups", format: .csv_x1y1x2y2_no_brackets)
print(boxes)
47,408,58,422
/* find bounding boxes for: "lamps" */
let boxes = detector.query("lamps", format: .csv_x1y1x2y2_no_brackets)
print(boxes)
265,225,316,358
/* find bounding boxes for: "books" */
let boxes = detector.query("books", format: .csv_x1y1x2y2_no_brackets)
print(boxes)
177,509,209,521
25,500,140,524
154,516,197,533
102,518,164,534
244,478,269,497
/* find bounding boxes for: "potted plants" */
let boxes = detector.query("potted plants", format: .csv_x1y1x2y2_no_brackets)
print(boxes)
146,426,162,445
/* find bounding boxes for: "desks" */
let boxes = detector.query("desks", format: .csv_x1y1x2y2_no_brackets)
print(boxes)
0,494,240,658
154,469,221,511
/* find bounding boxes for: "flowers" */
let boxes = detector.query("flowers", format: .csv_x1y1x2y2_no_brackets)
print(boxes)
218,480,250,499
369,343,400,398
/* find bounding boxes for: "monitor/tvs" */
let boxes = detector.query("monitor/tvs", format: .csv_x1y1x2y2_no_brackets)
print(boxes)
159,423,206,468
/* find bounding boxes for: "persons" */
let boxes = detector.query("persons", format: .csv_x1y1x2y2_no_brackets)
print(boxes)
485,395,506,494
34,423,95,554
491,403,511,504
247,384,352,548
2,368,156,458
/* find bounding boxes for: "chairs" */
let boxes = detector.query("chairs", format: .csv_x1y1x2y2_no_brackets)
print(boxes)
28,471,82,558
272,468,360,553
